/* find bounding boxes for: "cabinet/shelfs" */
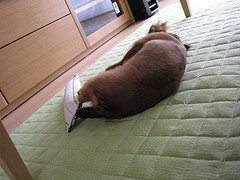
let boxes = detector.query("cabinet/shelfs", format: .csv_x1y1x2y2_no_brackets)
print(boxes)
0,0,136,120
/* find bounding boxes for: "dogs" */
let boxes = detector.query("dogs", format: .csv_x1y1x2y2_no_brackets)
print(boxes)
75,19,191,121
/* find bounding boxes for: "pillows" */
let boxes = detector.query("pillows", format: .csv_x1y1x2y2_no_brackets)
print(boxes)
63,73,83,133
